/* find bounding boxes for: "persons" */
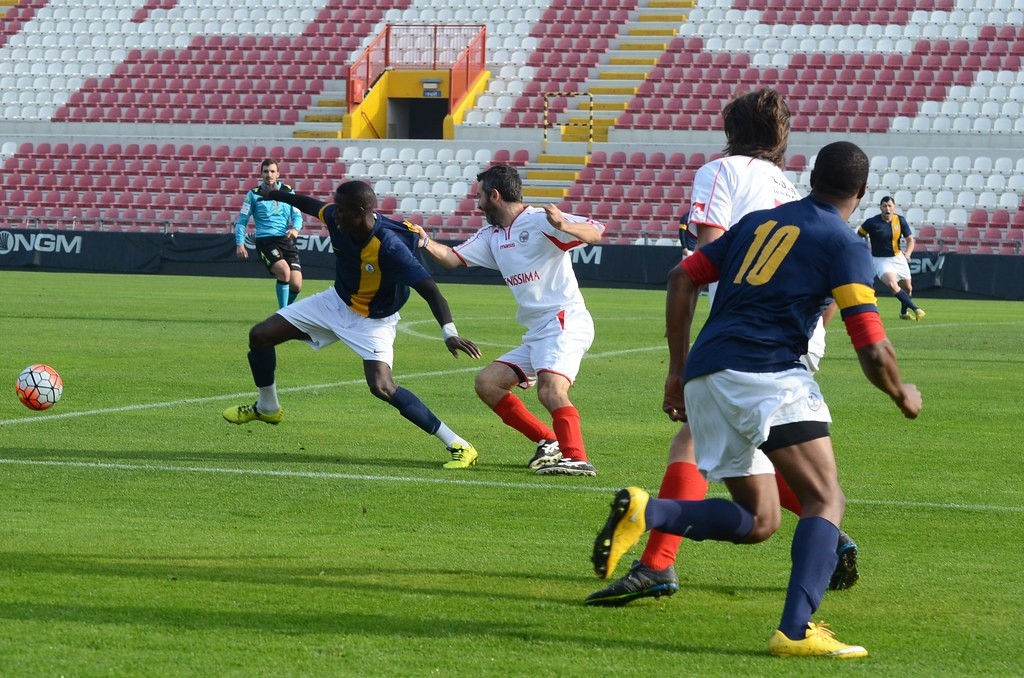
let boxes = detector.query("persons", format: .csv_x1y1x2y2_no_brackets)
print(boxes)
584,86,859,606
235,158,303,308
224,181,482,469
591,138,922,657
857,197,924,320
415,165,606,474
679,212,698,294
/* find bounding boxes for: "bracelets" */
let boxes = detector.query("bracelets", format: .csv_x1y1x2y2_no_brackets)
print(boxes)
422,234,429,248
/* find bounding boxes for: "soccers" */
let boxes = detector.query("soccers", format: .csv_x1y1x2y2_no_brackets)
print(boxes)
16,364,63,411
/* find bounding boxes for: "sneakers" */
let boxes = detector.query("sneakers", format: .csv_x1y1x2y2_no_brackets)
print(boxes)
583,559,679,606
535,458,597,477
222,401,283,425
590,486,649,579
827,531,860,590
528,439,563,470
442,440,479,469
768,619,868,657
899,311,916,320
915,309,925,321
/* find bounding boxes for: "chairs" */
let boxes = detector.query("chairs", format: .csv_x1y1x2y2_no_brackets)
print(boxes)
0,0,1024,255
